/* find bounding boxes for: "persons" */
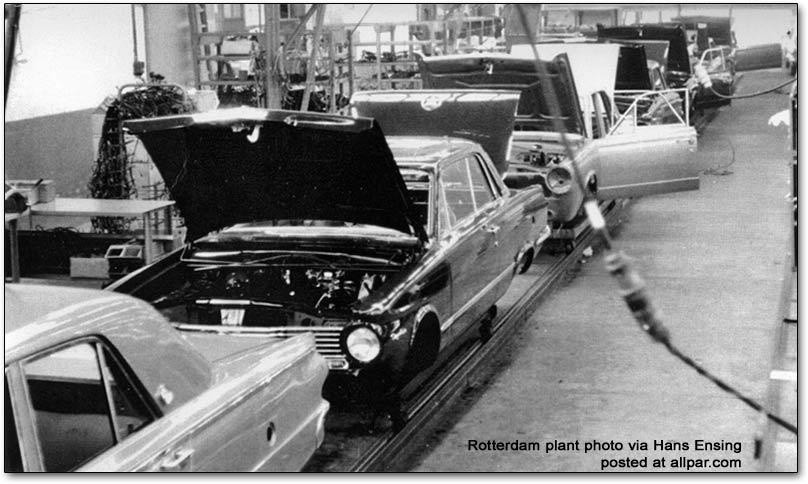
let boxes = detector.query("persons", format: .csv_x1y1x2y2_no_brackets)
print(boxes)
783,31,796,68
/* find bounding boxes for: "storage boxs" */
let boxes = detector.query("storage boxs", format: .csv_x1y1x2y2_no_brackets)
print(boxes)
5,179,56,202
68,252,110,279
5,185,40,206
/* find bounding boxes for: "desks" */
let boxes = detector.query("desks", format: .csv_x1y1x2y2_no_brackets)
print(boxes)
5,198,175,282
